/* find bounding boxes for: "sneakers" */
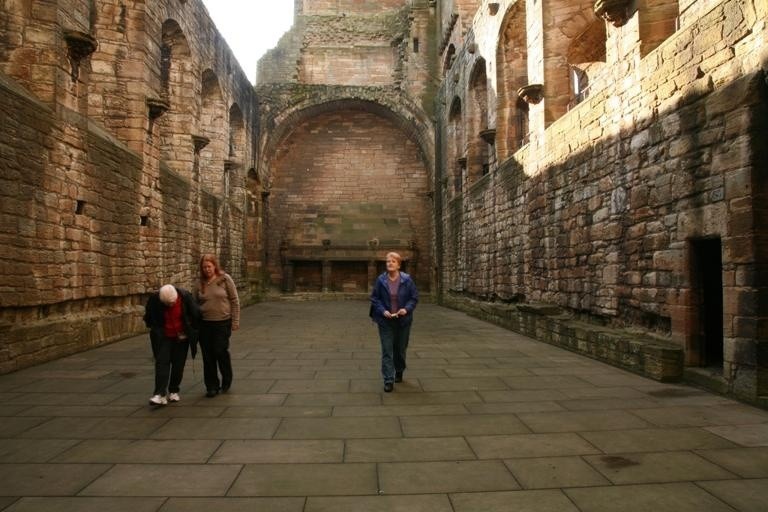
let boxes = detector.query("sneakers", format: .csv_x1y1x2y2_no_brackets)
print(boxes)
170,393,180,401
395,372,402,383
384,383,393,392
206,389,218,398
219,387,225,394
150,395,168,405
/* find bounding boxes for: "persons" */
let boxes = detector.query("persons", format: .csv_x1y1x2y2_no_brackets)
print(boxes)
142,284,200,406
192,253,240,398
367,251,418,392
